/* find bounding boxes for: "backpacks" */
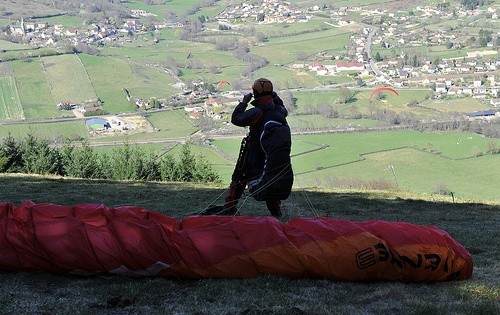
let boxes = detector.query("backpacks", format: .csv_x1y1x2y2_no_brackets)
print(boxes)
248,116,294,201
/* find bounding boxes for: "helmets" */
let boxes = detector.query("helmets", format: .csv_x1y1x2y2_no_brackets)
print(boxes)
251,78,274,94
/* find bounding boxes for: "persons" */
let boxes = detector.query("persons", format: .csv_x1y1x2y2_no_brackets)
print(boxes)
215,77,288,218
